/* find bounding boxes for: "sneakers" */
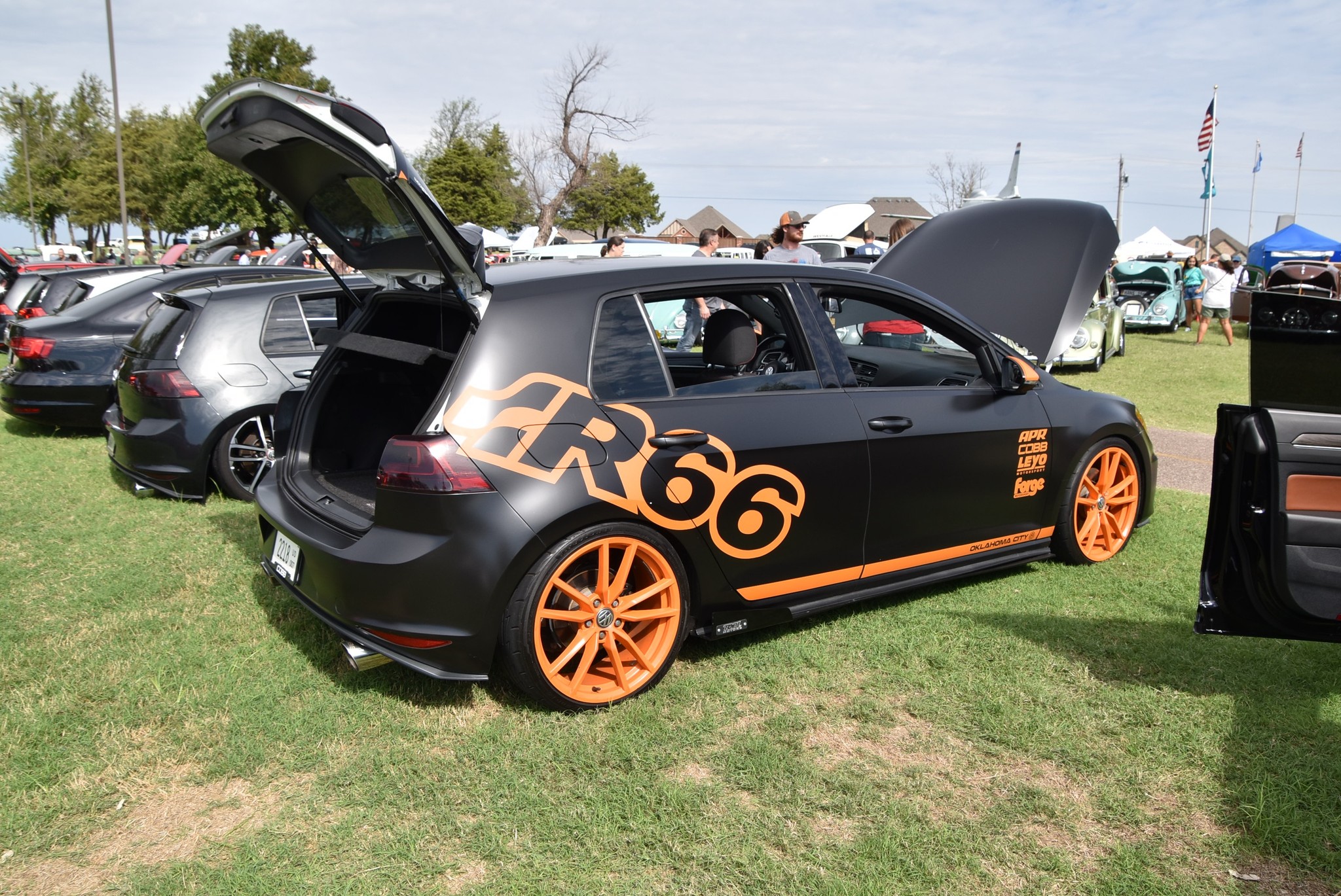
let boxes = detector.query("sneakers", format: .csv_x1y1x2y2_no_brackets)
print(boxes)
1185,327,1190,332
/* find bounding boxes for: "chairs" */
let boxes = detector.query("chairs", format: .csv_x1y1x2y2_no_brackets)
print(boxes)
696,308,758,385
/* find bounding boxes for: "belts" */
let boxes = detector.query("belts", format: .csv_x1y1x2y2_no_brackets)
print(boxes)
1184,284,1200,289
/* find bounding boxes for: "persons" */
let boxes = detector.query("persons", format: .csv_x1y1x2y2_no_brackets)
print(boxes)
888,217,916,249
674,228,726,352
853,229,885,254
239,250,252,265
1228,253,1249,322
308,238,326,271
1192,253,1237,346
1181,255,1207,332
762,211,823,266
600,236,625,257
56,249,125,265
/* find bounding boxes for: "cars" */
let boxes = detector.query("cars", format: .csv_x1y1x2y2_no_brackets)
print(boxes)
193,78,1158,716
1193,291,1341,648
0,204,891,358
0,267,349,440
101,267,390,507
1110,257,1188,333
991,269,1127,374
1231,260,1341,337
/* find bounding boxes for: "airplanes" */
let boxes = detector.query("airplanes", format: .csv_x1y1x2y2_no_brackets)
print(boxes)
933,142,1022,215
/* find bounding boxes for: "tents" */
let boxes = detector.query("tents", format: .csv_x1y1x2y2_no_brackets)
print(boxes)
463,221,513,263
1115,226,1196,262
1247,222,1341,273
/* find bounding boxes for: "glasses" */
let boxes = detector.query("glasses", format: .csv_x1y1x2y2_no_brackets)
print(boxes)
787,224,806,229
888,234,890,239
762,251,767,253
1233,261,1240,262
1188,255,1195,258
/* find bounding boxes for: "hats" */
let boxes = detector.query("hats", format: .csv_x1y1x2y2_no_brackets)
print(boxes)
1232,256,1241,262
780,211,810,225
1218,253,1231,263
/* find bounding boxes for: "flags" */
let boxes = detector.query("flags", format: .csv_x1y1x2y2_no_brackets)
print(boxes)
1296,137,1303,157
1199,144,1216,199
1252,153,1262,173
1198,98,1219,151
